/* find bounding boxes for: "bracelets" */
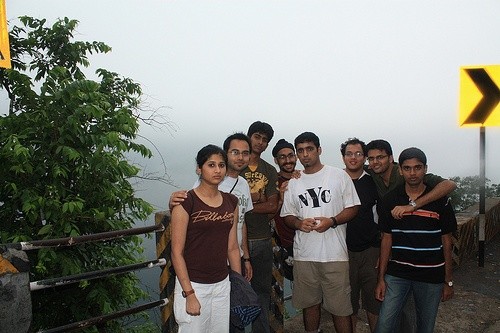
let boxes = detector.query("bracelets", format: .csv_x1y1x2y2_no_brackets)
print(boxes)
243,257,249,262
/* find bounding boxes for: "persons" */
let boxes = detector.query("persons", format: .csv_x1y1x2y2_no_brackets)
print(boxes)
272,139,298,281
171,144,241,333
278,132,362,333
238,121,279,333
362,140,455,220
292,139,381,333
169,132,253,282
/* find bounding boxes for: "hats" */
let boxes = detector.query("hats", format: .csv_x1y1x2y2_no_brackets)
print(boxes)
272,139,294,157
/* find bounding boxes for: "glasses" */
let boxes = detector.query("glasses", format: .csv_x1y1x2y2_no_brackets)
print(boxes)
344,152,365,157
366,155,388,160
277,154,295,159
228,151,249,156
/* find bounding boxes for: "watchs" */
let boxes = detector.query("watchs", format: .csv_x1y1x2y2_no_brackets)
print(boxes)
409,199,416,209
446,281,454,287
375,147,453,333
331,217,337,228
181,290,194,298
